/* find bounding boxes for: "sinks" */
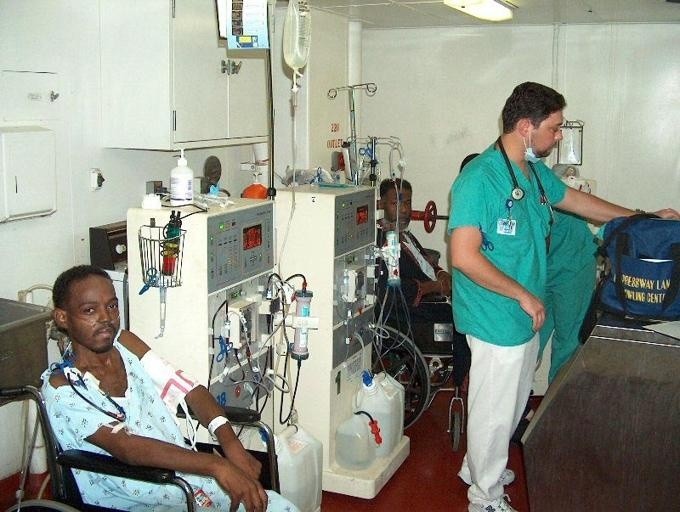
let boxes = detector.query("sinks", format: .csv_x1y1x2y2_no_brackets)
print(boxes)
2,297,53,385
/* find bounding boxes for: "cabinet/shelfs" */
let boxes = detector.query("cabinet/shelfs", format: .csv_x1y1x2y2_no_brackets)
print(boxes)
99,0,270,152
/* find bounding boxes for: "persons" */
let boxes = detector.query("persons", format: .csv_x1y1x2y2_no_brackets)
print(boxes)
374,177,474,388
38,263,304,512
455,153,601,441
446,80,679,512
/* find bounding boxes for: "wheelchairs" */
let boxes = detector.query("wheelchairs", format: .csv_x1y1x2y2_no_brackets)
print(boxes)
374,297,465,452
1,385,281,512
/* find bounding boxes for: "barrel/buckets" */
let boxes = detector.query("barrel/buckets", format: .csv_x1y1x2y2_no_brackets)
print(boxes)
335,414,373,469
353,371,405,456
275,424,322,512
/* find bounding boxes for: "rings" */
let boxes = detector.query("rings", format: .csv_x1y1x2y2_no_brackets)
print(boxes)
667,208,671,211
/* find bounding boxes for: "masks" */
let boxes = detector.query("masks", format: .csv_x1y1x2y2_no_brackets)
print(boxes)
522,135,541,164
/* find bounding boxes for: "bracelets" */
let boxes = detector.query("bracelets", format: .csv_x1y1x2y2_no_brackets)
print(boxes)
636,209,646,216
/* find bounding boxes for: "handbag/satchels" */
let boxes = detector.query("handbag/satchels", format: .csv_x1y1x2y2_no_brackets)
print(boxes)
596,213,680,321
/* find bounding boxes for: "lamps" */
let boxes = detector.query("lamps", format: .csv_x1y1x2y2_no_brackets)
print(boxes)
443,0,519,24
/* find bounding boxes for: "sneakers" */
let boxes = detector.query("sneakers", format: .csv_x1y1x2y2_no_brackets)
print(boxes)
456,451,522,512
